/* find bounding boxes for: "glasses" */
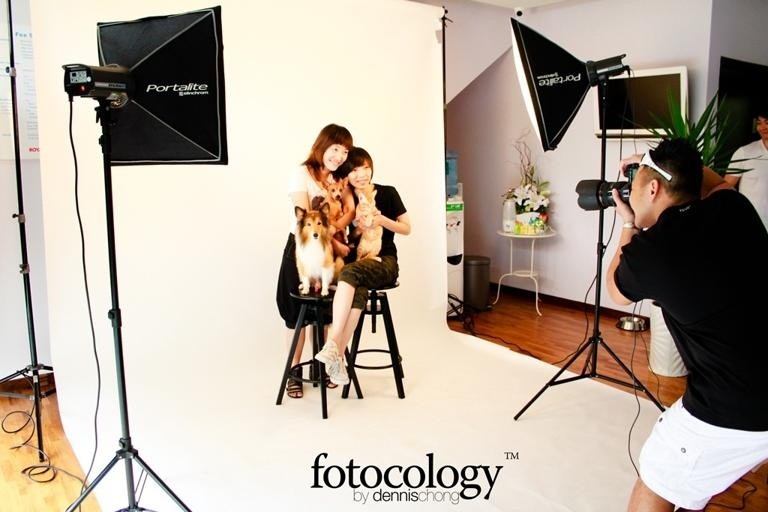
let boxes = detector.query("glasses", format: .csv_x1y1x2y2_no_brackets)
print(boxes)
638,148,672,181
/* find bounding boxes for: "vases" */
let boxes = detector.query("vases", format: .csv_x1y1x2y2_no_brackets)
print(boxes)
513,220,544,235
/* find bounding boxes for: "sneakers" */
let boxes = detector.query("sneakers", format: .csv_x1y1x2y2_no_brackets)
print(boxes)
315,340,341,365
325,354,350,385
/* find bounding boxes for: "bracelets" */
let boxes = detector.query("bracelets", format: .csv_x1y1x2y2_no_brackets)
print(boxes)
622,221,643,231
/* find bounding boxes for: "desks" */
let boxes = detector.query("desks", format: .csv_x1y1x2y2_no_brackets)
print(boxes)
491,227,556,315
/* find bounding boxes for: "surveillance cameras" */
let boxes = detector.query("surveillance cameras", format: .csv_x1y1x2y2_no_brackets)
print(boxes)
514,6,525,18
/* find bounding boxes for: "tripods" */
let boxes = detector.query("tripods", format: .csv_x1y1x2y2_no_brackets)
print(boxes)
66,98,192,512
513,77,666,420
441,7,491,337
0,0,57,461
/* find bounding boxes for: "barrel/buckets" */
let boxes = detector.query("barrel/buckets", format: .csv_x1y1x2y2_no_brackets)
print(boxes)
445,150,459,201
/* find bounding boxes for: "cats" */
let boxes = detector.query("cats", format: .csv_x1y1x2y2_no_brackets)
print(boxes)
352,189,383,263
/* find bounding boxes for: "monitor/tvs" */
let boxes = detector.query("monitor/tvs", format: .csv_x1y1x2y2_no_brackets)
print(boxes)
593,64,691,141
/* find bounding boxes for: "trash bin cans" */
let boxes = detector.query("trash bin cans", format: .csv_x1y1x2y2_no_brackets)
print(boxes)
464,255,492,313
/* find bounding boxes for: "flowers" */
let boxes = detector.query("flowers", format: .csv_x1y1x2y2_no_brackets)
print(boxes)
498,128,551,231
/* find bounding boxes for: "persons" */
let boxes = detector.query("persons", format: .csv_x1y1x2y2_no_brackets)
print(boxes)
722,113,768,234
606,130,768,512
313,145,411,386
276,123,354,399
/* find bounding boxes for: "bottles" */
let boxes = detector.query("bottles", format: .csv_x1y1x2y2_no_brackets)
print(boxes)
502,199,517,233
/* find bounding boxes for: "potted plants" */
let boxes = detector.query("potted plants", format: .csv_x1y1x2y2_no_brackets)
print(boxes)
619,84,768,378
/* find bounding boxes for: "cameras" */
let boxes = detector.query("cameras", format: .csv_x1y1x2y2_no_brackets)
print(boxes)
575,163,640,211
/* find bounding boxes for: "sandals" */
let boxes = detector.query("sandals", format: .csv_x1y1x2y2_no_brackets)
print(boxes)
286,367,304,398
310,365,339,388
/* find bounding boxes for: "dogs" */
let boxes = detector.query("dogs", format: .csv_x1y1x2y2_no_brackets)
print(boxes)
294,202,345,297
318,177,349,245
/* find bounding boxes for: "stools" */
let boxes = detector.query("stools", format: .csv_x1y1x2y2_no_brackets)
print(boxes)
275,285,363,418
339,279,406,400
